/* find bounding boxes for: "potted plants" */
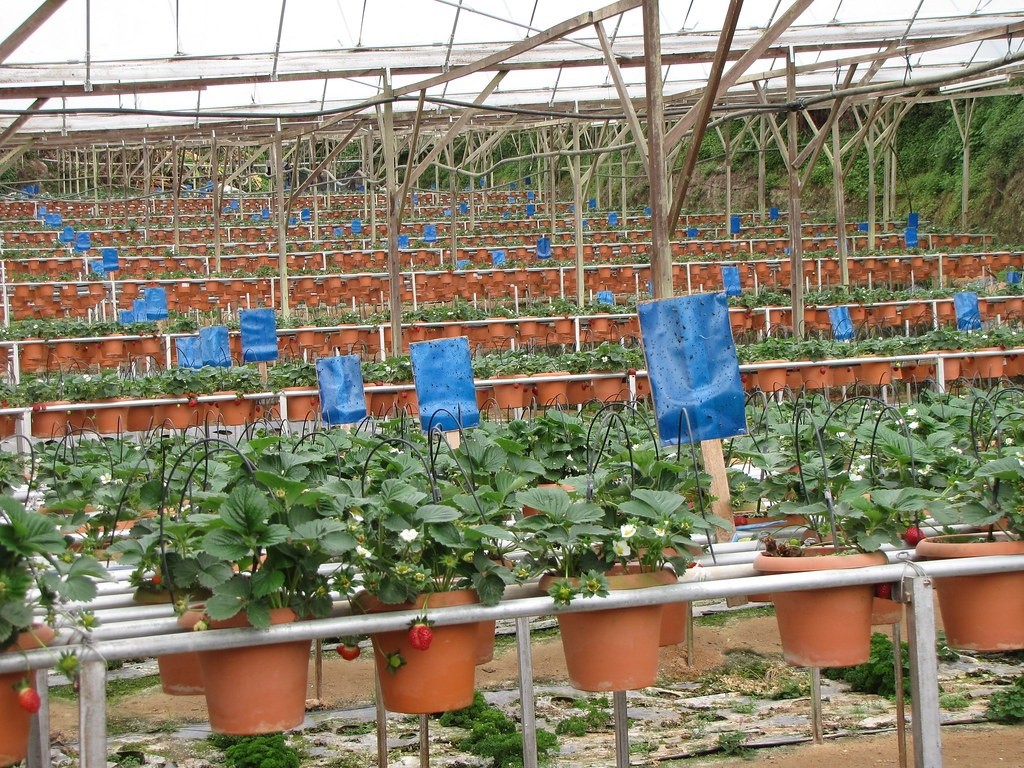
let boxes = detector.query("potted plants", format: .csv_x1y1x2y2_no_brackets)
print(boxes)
4,181,1024,768
